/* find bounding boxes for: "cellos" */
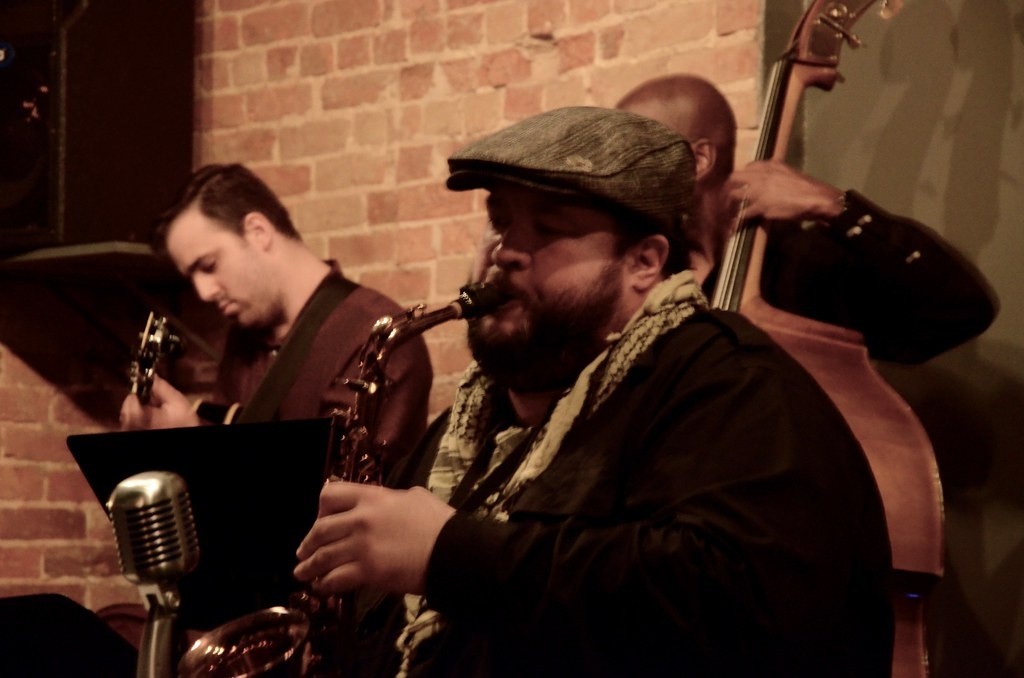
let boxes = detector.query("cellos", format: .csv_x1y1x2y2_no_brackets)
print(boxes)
707,1,952,678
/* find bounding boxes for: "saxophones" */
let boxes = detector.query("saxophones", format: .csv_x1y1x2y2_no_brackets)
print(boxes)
171,280,512,676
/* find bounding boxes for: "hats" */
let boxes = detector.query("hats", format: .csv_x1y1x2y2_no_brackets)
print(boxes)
446,107,696,229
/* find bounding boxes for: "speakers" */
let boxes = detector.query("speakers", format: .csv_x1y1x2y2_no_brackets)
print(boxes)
0,0,197,249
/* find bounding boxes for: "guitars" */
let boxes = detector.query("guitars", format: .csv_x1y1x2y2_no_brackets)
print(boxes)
126,311,182,404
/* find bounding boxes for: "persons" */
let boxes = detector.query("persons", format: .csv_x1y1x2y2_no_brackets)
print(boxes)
615,76,999,366
291,104,894,677
117,163,433,678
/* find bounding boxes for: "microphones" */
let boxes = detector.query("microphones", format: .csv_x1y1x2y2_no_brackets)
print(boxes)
106,471,201,678
